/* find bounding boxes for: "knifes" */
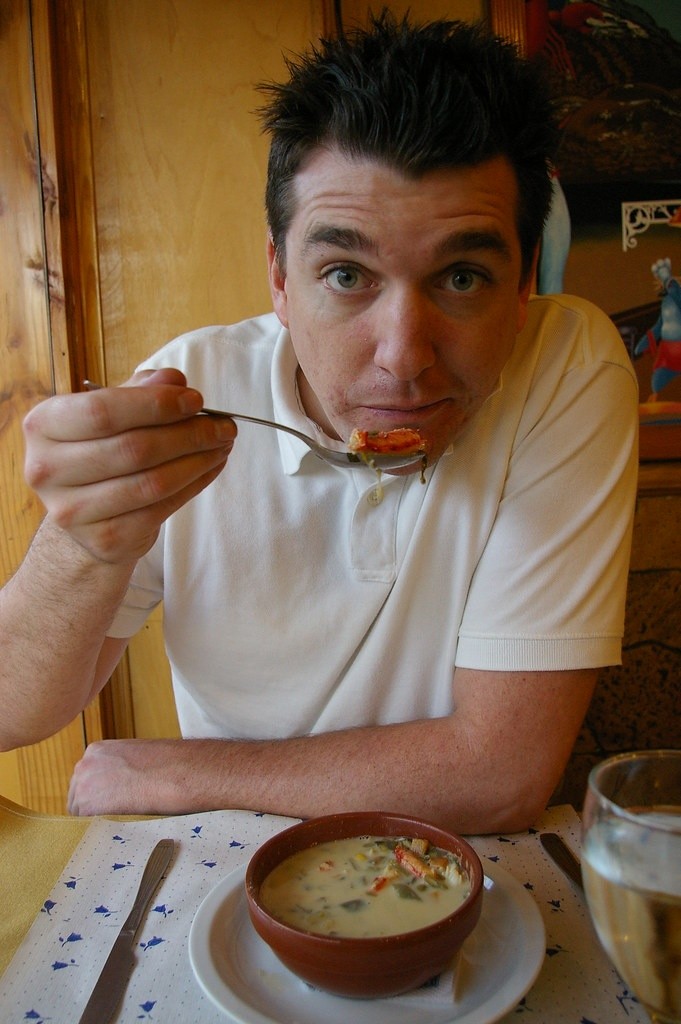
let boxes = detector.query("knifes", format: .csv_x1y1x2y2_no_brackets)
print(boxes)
539,833,586,907
78,839,174,1024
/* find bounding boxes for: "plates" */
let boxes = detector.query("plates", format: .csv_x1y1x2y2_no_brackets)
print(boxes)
188,853,545,1024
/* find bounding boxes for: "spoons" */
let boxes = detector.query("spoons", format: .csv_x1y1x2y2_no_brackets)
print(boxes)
83,378,426,470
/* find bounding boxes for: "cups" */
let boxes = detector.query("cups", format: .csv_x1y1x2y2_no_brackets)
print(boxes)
580,749,681,1024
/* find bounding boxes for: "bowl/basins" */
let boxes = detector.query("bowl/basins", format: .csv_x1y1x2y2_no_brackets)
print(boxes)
246,811,484,999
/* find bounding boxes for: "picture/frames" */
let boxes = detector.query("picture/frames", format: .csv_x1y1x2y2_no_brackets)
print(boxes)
490,0,681,462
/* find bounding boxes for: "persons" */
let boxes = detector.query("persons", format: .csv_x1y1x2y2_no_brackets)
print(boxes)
0,15,639,837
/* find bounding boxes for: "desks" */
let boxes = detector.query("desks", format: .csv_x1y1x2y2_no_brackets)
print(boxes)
0,796,681,1024
